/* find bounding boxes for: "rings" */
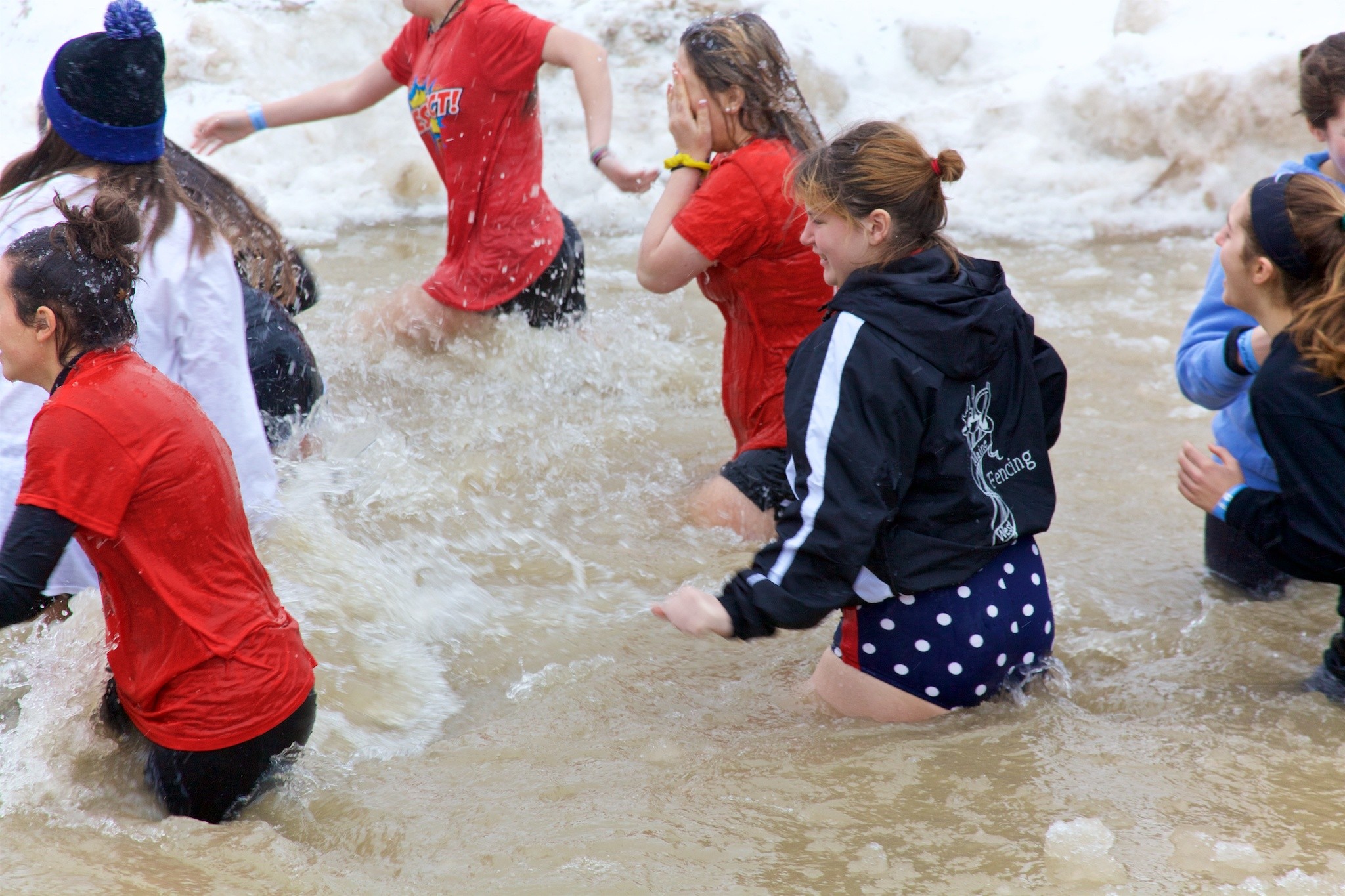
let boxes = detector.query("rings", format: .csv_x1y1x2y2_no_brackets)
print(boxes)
635,176,645,186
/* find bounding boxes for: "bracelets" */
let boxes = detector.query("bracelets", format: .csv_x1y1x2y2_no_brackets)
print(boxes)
1210,483,1250,522
589,144,611,166
1235,327,1265,376
664,152,712,176
246,104,268,132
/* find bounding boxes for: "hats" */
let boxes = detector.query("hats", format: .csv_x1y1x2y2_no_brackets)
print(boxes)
41,0,167,164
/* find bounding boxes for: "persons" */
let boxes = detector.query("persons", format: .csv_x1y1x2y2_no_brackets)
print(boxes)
647,116,1070,728
187,3,663,362
1174,170,1344,710
1173,31,1344,598
0,0,283,628
636,14,837,544
2,188,320,828
